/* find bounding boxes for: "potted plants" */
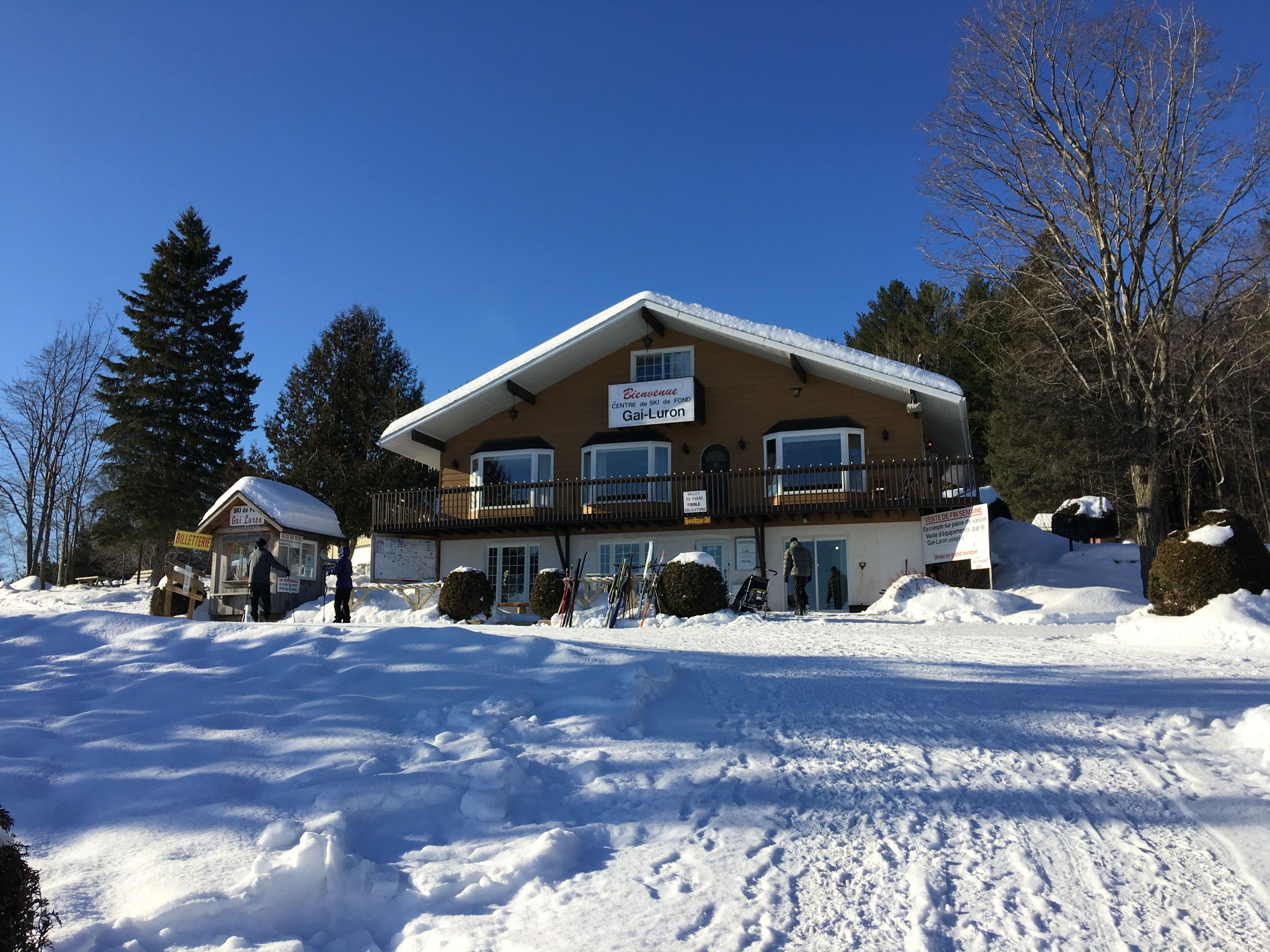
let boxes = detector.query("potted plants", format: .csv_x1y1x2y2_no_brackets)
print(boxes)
530,568,573,627
438,566,494,625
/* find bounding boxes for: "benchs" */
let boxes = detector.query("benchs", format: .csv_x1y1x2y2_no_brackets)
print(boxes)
497,602,529,613
75,576,105,585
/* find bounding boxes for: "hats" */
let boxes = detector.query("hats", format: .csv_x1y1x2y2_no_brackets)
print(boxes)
255,537,267,547
790,537,798,542
830,566,837,570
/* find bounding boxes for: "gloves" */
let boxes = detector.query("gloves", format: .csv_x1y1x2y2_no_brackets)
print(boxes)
783,577,790,583
806,576,812,583
285,568,291,577
825,599,830,604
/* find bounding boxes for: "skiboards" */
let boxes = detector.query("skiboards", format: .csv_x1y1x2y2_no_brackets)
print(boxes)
562,551,588,628
602,540,665,629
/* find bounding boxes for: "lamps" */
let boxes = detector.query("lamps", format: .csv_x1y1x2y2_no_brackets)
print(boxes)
642,321,653,352
906,393,923,413
681,442,690,453
881,428,889,441
451,457,459,469
507,393,520,425
738,438,746,450
925,438,933,450
790,368,802,402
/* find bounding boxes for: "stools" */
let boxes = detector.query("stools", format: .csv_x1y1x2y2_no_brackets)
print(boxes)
872,489,889,501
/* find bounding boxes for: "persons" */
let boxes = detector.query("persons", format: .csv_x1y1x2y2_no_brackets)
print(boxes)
326,545,353,623
783,537,814,616
246,538,291,623
826,566,848,610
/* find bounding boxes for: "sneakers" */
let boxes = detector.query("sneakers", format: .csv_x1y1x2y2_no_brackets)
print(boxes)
804,605,809,614
333,616,341,623
795,609,803,615
342,616,351,622
251,618,258,622
263,618,269,622
